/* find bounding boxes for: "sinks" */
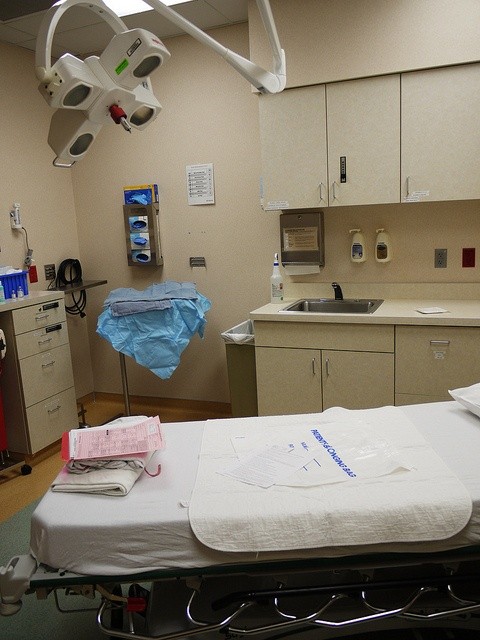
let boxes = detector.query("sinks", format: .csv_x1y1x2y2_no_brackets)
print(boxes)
283,298,384,314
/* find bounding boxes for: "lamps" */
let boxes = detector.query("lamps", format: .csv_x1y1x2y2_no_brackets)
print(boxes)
34,0,286,167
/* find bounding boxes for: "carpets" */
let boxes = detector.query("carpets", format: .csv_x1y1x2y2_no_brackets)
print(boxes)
0,490,156,640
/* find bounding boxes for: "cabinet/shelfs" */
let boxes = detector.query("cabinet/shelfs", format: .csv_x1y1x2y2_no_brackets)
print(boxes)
396,325,480,408
253,320,394,417
0,291,80,455
261,73,400,213
401,60,480,204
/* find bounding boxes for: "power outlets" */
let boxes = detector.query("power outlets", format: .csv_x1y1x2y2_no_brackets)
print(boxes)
435,249,447,268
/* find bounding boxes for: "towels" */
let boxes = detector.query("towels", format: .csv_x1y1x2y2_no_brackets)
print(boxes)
189,406,473,553
65,453,148,475
50,416,157,496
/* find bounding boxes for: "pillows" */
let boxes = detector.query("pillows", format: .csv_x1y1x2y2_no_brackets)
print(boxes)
447,381,480,418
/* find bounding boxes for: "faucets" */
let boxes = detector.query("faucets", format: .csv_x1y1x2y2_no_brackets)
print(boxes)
331,282,343,300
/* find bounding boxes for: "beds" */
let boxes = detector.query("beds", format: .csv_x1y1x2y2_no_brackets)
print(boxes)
0,399,480,639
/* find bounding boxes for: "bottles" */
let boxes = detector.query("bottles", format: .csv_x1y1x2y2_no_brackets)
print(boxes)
11,290,16,301
17,286,23,298
